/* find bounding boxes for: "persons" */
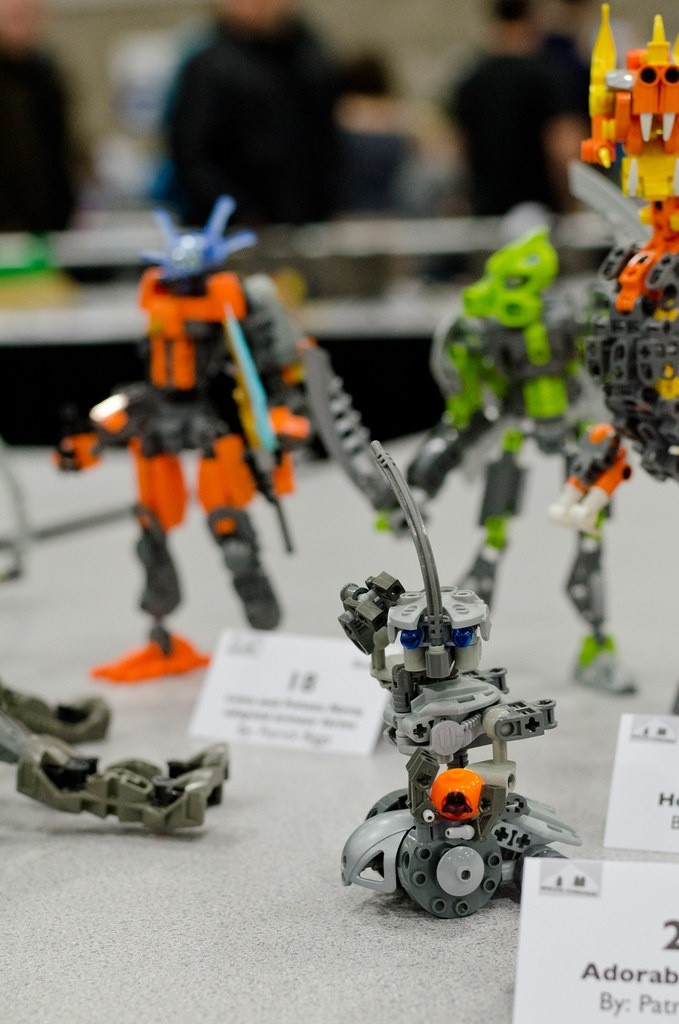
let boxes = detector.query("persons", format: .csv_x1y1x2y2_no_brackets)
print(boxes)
375,227,634,694
161,0,420,227
580,4,679,310
0,1,79,236
53,200,310,685
442,0,621,222
